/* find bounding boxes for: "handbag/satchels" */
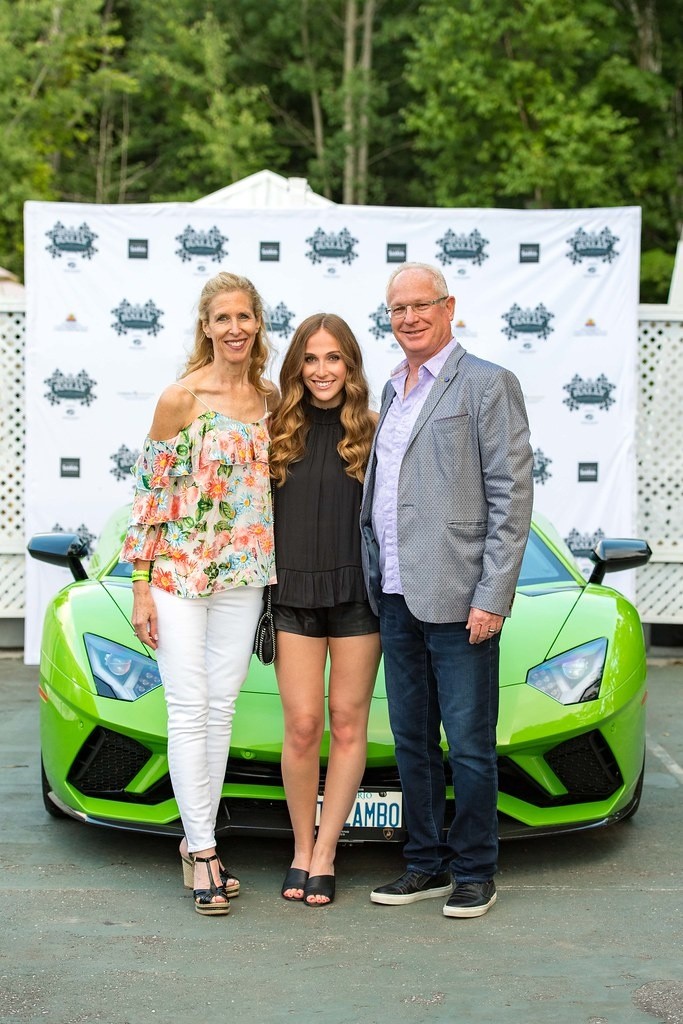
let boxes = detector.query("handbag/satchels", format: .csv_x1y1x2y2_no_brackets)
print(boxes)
253,612,276,666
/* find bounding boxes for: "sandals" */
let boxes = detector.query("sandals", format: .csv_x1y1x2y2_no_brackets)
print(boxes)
282,868,309,901
304,874,336,907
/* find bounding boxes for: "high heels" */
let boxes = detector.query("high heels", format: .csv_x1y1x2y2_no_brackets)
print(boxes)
189,852,230,915
178,831,240,898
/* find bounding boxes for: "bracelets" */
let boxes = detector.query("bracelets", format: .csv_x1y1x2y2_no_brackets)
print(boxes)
131,570,150,582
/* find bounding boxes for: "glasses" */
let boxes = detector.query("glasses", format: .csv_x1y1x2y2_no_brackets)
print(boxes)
386,296,447,319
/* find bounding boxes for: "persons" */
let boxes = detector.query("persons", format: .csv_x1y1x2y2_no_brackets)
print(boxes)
358,263,532,918
268,314,380,906
123,274,379,915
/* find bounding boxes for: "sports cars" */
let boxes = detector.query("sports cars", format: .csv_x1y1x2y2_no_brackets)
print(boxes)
27,502,653,846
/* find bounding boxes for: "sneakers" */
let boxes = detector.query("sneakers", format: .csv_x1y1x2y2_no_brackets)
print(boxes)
370,867,453,905
443,880,498,918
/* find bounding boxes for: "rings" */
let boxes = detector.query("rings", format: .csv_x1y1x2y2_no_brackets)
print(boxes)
489,630,496,633
